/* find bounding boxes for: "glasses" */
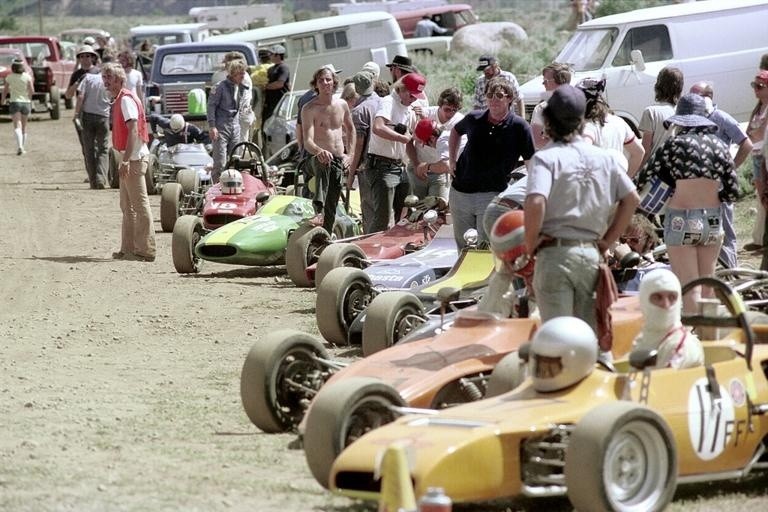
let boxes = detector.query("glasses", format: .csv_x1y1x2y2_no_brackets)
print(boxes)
618,235,650,247
543,79,555,84
484,92,509,101
749,81,767,91
441,106,458,113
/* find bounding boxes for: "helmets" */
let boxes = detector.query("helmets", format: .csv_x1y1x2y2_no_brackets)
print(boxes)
527,317,599,392
219,169,244,194
690,82,713,101
489,211,536,279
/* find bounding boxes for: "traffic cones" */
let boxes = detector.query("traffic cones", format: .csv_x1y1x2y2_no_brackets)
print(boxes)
379,443,418,512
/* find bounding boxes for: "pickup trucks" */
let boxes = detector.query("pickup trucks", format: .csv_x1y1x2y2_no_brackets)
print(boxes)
0,37,77,110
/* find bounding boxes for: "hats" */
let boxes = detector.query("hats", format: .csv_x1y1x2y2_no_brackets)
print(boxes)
547,84,586,130
353,71,374,96
83,32,110,47
477,54,496,71
220,51,247,65
385,55,414,73
663,92,720,133
415,119,437,149
320,64,343,75
258,45,286,57
401,73,427,100
76,45,100,63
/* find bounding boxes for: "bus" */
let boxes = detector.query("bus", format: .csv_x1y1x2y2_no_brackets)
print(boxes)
206,12,409,92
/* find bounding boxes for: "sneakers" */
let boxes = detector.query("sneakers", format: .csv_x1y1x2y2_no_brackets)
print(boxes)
112,252,156,262
308,213,325,227
17,149,23,155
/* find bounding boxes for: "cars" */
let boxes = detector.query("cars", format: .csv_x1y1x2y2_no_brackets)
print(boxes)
404,36,451,56
262,87,343,161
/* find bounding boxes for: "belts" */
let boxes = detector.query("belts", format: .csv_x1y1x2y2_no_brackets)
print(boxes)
491,196,523,210
533,240,595,256
368,155,405,167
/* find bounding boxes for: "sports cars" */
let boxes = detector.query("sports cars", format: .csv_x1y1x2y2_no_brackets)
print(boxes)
0,48,60,119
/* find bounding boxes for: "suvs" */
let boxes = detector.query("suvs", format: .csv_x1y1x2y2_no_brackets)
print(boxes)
126,22,207,82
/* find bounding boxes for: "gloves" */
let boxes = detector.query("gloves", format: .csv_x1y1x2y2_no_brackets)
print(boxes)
409,196,440,223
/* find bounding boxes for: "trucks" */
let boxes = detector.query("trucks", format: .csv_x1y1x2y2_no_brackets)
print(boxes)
519,0,768,139
329,0,448,16
188,3,283,32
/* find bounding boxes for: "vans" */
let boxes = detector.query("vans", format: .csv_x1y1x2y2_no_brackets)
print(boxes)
396,4,478,38
58,30,111,46
144,43,257,142
